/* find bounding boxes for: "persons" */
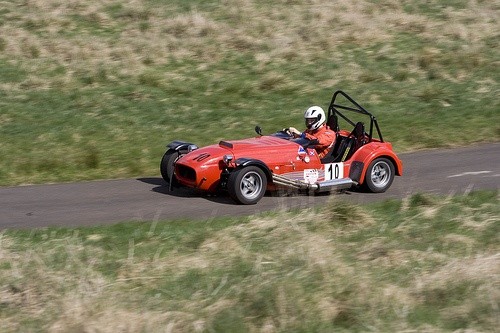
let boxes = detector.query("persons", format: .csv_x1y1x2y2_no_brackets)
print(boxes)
289,105,336,159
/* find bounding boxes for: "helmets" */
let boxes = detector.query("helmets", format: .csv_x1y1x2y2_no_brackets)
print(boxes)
304,106,326,131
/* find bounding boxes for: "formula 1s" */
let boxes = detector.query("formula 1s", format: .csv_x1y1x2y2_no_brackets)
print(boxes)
160,89,403,205
